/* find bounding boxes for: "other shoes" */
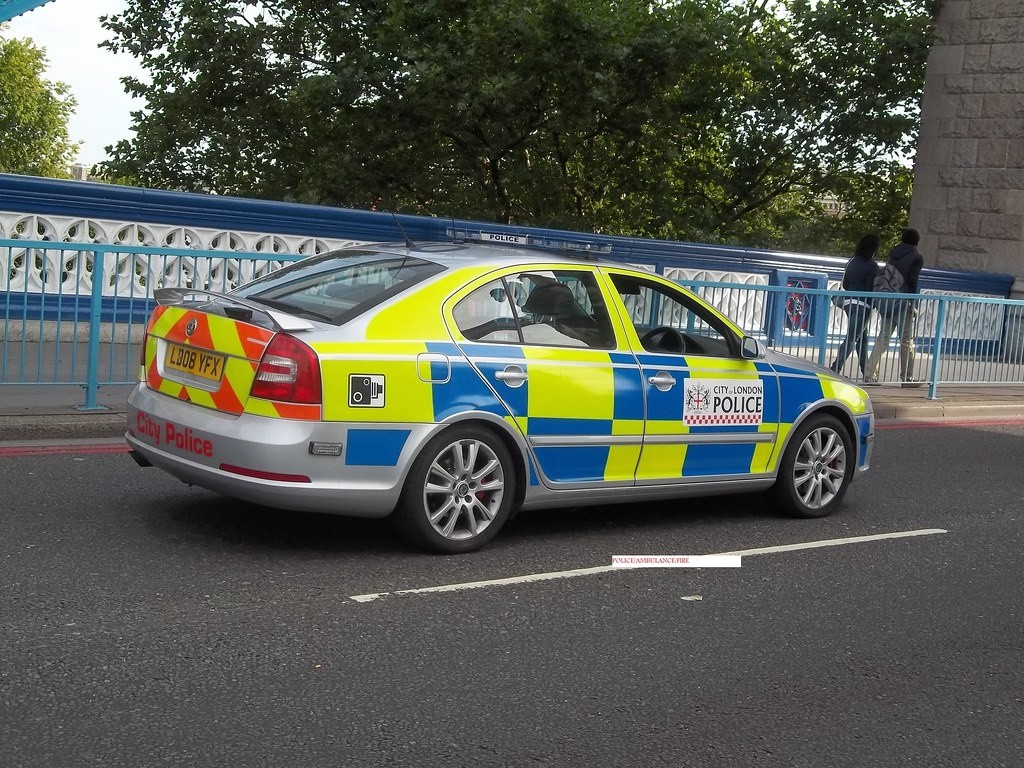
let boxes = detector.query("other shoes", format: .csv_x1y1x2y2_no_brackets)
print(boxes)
865,381,882,386
901,378,926,388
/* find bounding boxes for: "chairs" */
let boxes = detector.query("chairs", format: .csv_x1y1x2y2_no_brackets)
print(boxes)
520,282,580,340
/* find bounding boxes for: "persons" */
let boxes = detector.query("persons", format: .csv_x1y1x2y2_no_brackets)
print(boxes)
829,233,879,380
863,229,927,388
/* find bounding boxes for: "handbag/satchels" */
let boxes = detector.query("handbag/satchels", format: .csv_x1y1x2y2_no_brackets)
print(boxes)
831,285,845,310
873,265,907,317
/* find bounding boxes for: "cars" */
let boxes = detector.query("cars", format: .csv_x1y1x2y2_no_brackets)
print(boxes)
124,241,875,555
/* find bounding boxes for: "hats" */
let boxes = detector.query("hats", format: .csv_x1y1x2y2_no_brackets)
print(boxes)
902,229,919,246
